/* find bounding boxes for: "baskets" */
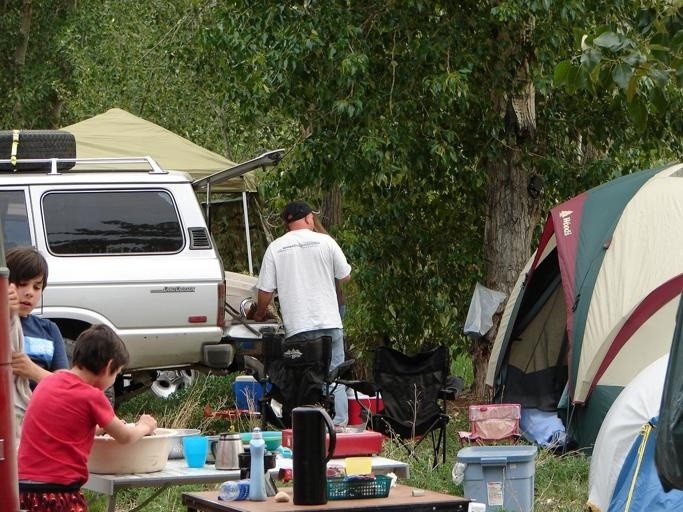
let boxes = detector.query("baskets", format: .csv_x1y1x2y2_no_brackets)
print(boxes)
326,474,393,500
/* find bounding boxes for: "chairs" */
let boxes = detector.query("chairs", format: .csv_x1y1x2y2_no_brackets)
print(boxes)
365,344,455,473
456,403,521,448
257,334,354,432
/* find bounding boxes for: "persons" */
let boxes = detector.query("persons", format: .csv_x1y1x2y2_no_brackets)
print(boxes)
311,208,351,320
5,248,69,391
254,203,351,427
18,327,157,512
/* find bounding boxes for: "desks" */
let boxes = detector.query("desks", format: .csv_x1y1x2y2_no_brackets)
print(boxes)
178,486,470,512
80,454,409,512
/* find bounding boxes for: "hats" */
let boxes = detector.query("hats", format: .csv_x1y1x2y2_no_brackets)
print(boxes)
280,199,321,224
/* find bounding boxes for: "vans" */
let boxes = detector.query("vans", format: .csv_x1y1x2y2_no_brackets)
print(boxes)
0,149,283,412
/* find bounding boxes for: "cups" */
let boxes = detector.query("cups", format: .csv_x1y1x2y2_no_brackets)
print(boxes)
344,457,374,477
293,405,337,506
183,436,209,468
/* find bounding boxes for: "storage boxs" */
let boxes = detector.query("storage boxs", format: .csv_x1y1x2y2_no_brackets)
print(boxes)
346,396,383,426
234,375,272,412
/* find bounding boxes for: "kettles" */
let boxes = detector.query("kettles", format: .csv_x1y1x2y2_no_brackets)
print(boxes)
212,434,245,471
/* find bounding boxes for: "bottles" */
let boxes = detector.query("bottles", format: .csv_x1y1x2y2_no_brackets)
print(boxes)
249,427,267,501
220,479,250,501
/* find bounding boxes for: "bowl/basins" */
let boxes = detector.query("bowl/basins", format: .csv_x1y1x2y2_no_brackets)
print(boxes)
241,432,282,451
169,429,202,458
87,428,180,474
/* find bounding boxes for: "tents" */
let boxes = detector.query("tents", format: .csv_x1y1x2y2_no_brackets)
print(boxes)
55,108,257,274
487,164,682,451
587,351,683,512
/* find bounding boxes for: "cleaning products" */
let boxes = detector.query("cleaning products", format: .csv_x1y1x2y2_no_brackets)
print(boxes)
250,427,269,503
